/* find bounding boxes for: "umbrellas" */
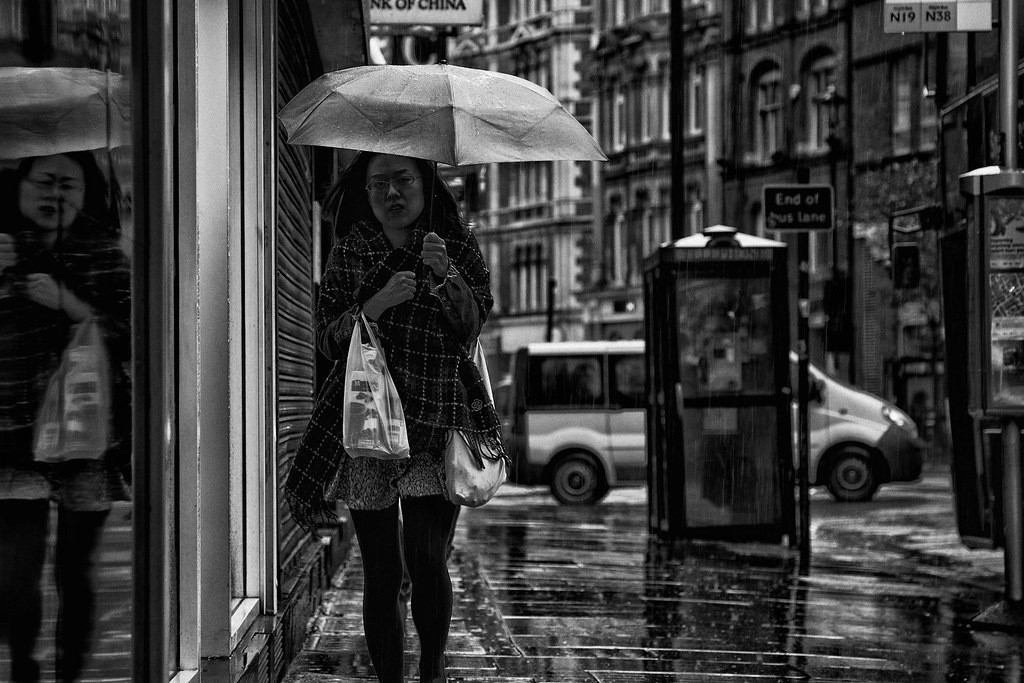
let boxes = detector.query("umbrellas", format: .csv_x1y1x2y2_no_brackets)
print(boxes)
0,63,130,160
277,63,608,169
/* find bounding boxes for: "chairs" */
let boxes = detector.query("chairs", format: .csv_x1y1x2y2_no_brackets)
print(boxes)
571,363,604,405
546,368,571,406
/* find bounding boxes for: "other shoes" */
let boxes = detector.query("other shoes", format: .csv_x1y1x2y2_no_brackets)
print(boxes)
418,651,449,683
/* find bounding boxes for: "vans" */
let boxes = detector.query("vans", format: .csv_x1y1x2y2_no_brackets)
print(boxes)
508,340,926,510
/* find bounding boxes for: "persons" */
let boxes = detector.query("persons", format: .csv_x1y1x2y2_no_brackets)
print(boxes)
570,363,594,403
0,151,129,683
283,150,500,683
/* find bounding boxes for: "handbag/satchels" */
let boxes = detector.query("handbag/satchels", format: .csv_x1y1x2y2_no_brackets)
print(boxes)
32,313,108,461
444,339,509,505
343,311,411,459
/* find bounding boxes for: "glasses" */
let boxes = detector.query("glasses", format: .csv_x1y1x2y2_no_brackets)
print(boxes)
20,173,87,198
366,174,423,194
